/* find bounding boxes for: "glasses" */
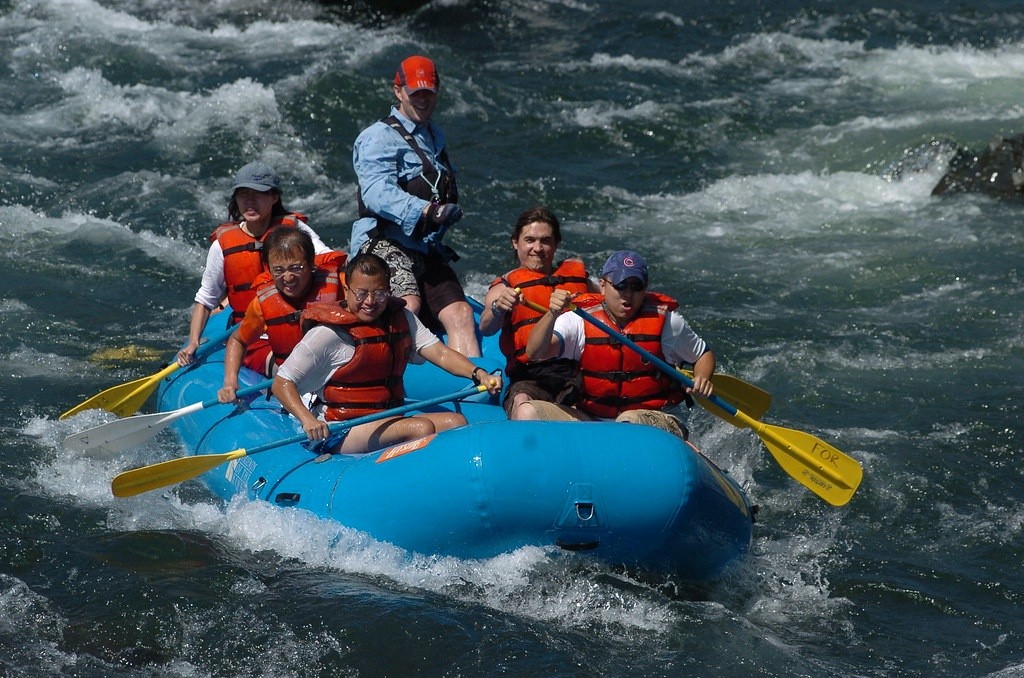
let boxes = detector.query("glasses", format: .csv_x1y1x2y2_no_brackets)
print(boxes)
346,283,390,303
604,277,644,291
267,264,311,275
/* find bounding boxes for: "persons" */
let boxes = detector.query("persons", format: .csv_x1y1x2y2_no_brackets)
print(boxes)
177,161,714,456
351,56,480,358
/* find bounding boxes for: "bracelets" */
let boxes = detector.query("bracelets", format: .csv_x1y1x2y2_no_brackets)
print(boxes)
472,367,487,385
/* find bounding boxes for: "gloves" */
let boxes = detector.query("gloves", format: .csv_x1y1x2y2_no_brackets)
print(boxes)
426,202,463,225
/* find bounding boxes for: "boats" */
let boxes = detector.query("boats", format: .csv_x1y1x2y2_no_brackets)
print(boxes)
156,295,762,598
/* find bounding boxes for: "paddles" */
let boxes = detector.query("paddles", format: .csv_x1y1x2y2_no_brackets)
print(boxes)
58,319,243,422
562,289,863,507
87,344,180,369
512,286,770,429
436,223,448,242
63,378,276,460
109,379,495,498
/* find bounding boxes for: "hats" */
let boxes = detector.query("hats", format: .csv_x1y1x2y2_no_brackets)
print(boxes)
601,250,648,286
231,163,281,193
393,55,439,95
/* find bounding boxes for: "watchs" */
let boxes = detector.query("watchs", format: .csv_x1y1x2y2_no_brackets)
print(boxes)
490,300,502,314
